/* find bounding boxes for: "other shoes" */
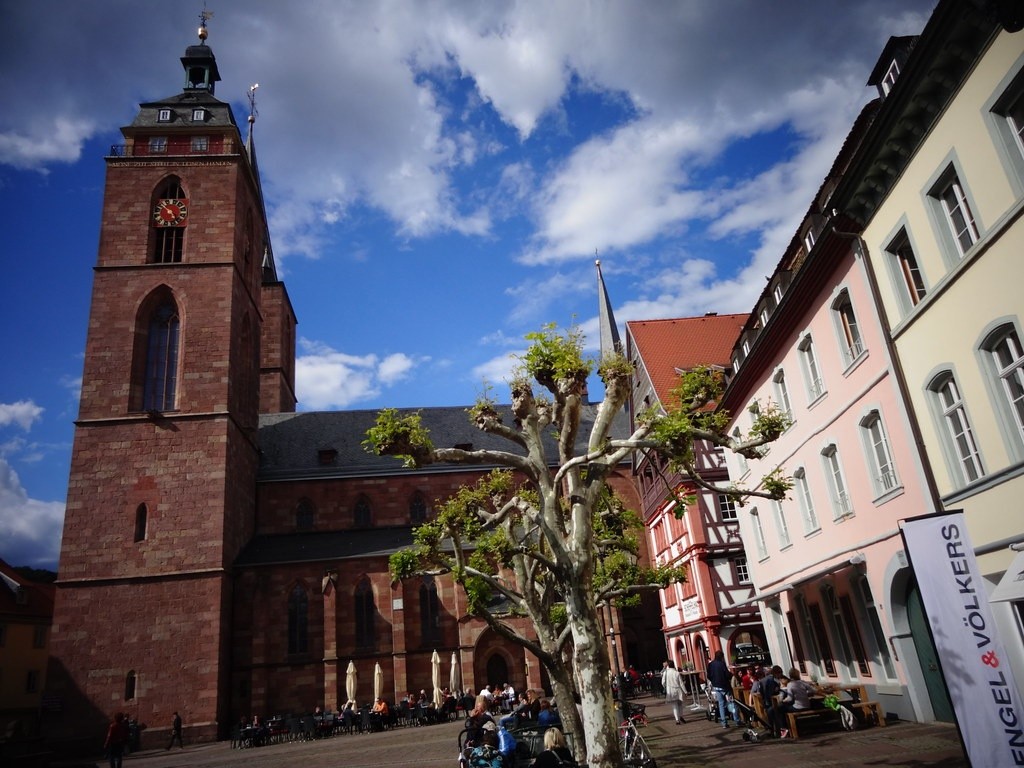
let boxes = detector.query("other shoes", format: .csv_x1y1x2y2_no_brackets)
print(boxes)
780,729,789,739
679,717,686,724
736,722,746,726
676,721,680,725
723,725,730,728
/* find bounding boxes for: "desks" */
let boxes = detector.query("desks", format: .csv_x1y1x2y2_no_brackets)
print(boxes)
732,683,872,736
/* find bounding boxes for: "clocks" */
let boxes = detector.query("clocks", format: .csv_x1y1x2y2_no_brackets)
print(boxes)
151,198,188,226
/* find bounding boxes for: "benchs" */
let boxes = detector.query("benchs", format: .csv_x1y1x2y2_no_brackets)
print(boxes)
787,700,886,739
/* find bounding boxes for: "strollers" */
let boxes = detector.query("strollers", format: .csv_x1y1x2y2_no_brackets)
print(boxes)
733,698,781,744
457,727,508,768
703,683,734,723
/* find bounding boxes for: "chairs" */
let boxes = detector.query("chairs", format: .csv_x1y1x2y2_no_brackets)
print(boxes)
228,694,508,751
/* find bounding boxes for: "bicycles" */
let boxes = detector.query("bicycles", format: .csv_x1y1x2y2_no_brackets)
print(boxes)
617,713,657,768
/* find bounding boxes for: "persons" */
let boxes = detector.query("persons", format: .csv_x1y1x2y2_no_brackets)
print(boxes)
661,660,688,725
608,664,639,738
235,683,515,751
103,712,130,768
462,687,583,768
707,650,744,728
164,711,184,751
730,664,828,738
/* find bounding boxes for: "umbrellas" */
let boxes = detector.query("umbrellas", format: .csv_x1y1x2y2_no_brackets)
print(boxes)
450,652,460,698
431,649,444,709
374,662,383,707
343,660,357,713
